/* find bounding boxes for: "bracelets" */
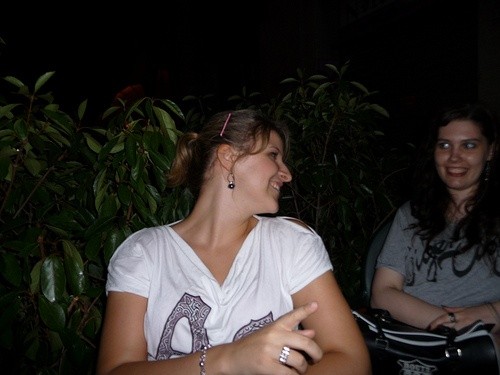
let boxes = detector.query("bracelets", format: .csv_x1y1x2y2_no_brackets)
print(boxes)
199,344,212,375
486,302,497,315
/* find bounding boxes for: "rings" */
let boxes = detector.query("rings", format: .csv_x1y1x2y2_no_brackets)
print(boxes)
279,347,290,365
448,312,457,323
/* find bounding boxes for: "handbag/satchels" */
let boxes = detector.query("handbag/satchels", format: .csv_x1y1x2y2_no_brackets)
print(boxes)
352,309,500,375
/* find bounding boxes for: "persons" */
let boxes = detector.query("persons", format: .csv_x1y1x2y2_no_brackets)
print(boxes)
370,107,500,353
97,109,371,375
